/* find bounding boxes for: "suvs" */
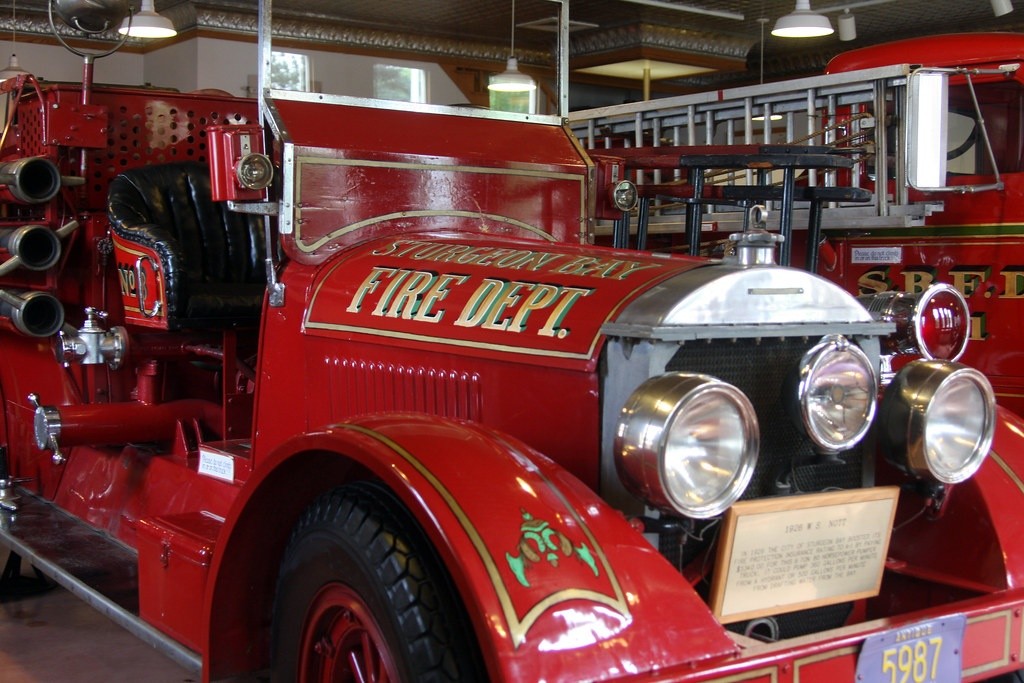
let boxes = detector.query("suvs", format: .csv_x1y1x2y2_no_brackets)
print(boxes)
0,0,1024,683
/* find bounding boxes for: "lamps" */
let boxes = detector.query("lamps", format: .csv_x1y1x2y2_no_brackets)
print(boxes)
488,1,536,92
118,0,177,38
0,0,30,78
990,0,1014,18
838,8,856,41
771,0,834,38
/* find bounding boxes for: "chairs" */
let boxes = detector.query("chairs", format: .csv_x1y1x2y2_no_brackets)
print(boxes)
108,160,290,331
